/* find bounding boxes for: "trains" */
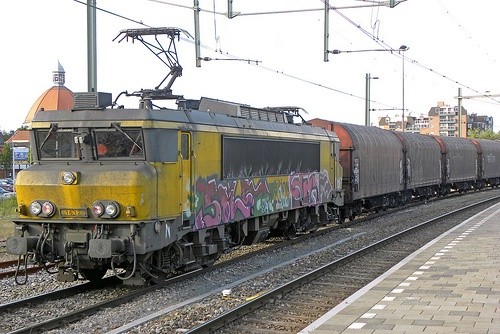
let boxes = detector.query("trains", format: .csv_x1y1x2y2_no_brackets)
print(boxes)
5,91,500,287
